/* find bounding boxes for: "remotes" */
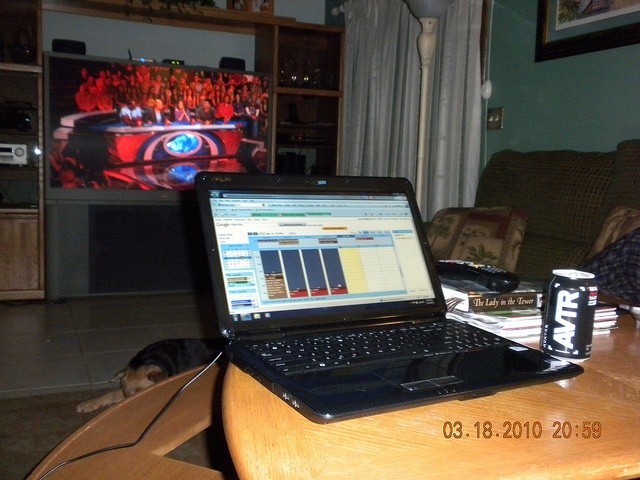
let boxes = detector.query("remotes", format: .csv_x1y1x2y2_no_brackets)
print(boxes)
435,258,487,294
451,260,520,291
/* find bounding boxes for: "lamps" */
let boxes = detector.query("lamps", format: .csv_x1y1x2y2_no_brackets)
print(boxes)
408,0,451,216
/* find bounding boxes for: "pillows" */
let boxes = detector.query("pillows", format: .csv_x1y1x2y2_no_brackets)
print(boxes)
427,207,473,266
443,207,530,273
587,207,640,259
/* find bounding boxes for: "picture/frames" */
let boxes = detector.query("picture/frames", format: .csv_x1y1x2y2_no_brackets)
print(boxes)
533,3,637,62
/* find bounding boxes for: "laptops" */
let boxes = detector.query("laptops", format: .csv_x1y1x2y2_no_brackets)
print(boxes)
196,171,583,425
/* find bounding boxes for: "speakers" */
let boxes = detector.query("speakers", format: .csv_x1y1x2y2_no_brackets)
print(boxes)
219,57,247,70
51,38,86,53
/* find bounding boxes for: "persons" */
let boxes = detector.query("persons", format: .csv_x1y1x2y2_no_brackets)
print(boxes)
71,64,268,152
578,226,638,308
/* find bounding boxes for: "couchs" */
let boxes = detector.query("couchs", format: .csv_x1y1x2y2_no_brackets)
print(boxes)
423,139,640,301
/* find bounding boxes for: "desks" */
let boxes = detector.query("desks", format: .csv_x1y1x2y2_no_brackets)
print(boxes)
28,288,639,478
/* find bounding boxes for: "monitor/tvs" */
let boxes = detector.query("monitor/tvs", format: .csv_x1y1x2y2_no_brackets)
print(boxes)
42,50,270,202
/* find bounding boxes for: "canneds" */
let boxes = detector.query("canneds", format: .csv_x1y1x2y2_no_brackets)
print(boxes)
538,269,599,365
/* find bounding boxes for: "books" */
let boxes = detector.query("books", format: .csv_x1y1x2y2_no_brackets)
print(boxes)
434,278,614,345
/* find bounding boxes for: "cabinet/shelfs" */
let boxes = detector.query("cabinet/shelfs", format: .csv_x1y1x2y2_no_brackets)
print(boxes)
0,0,44,299
44,0,297,297
255,22,346,175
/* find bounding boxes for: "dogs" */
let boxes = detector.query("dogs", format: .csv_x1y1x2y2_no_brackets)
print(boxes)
73,336,220,415
